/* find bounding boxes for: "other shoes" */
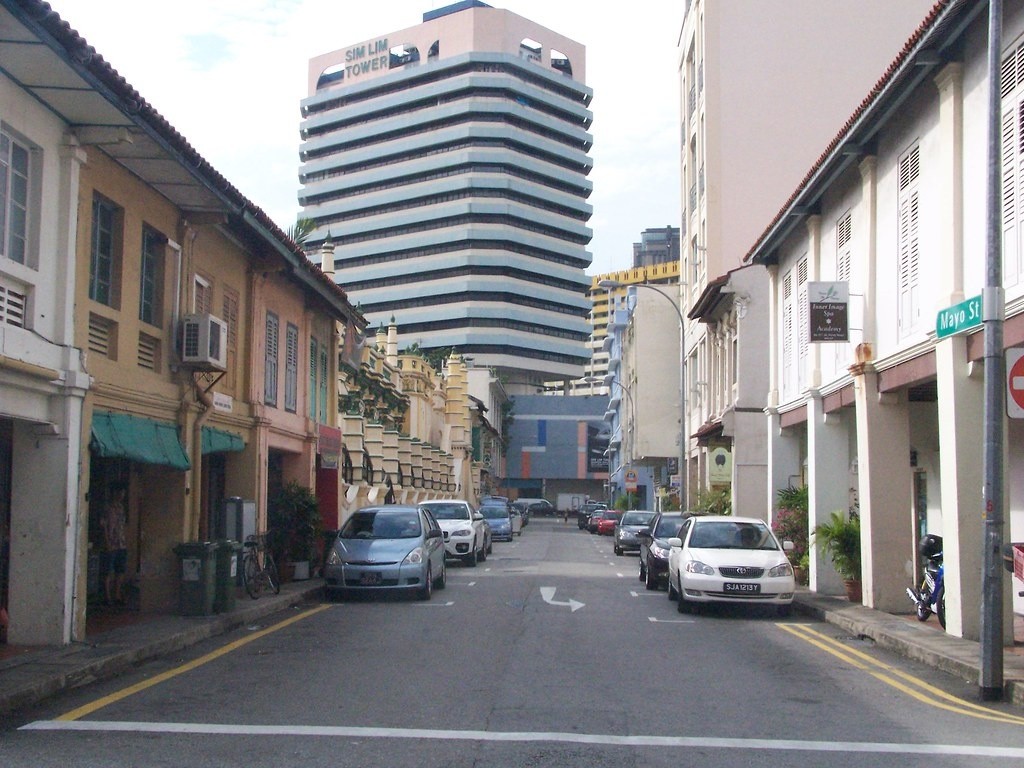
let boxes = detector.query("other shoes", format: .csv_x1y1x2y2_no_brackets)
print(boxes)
103,598,128,606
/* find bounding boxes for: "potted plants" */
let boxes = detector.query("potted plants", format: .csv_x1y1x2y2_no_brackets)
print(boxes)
794,555,809,586
271,478,325,580
807,510,863,602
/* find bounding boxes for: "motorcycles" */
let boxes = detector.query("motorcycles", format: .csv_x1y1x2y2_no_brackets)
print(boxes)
904,533,945,628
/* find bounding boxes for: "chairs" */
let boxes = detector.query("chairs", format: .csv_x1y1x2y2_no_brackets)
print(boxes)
660,522,676,537
732,528,754,548
697,529,715,547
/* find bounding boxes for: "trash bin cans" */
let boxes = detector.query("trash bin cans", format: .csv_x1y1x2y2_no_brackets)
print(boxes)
210,539,244,613
172,541,220,616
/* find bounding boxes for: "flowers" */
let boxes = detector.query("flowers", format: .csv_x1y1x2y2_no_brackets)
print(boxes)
769,507,805,563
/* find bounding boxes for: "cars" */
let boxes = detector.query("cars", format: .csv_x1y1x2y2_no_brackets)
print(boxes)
587,510,608,534
636,510,720,589
479,506,513,542
407,499,489,568
475,510,494,558
666,515,797,618
322,505,450,602
484,499,530,537
598,510,629,535
614,510,661,556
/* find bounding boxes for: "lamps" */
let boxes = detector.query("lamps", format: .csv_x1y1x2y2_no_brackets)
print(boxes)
969,361,984,378
779,427,794,438
878,393,899,405
824,412,841,426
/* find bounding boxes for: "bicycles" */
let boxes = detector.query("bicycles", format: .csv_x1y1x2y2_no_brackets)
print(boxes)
242,528,282,599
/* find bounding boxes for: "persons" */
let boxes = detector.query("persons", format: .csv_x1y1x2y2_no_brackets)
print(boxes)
100,486,128,605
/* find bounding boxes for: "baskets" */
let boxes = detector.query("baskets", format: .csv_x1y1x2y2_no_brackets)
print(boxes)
251,534,272,550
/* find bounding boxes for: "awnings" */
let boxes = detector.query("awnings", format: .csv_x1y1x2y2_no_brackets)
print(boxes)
91,411,243,476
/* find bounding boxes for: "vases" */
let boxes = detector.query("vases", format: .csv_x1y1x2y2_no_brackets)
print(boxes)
788,557,799,566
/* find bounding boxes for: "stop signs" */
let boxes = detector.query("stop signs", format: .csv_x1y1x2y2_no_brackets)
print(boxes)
1004,344,1024,421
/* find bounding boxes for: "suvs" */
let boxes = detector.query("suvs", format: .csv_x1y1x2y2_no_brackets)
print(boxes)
577,503,606,529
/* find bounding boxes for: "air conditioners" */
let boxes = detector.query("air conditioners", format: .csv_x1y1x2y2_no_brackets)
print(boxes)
182,311,229,368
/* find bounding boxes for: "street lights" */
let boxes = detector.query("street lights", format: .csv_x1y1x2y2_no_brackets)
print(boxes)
597,277,686,514
583,376,635,511
